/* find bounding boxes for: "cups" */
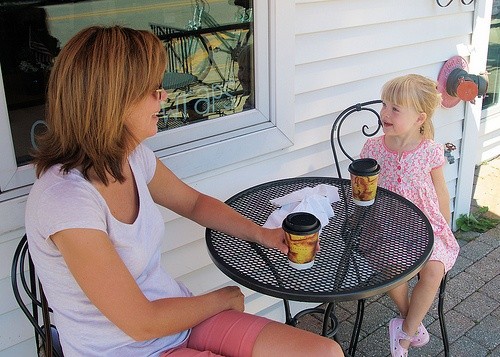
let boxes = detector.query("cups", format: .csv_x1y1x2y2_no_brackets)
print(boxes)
187,98,208,120
348,158,381,207
282,212,322,270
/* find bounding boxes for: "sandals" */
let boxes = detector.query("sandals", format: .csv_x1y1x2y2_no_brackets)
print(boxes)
399,312,431,348
388,318,415,357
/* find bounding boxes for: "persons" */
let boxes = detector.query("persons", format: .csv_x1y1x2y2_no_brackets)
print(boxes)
238,44,254,111
350,74,460,357
24,24,344,356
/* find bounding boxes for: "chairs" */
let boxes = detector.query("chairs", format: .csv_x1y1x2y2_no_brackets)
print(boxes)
11,233,65,357
330,99,449,357
157,54,236,133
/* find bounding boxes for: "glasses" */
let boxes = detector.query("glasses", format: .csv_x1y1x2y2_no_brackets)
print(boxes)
152,81,164,101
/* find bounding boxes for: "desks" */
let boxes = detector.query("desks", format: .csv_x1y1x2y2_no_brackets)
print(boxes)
162,72,196,123
207,177,434,357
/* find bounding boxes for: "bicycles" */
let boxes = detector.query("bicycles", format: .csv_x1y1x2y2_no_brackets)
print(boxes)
148,0,253,86
